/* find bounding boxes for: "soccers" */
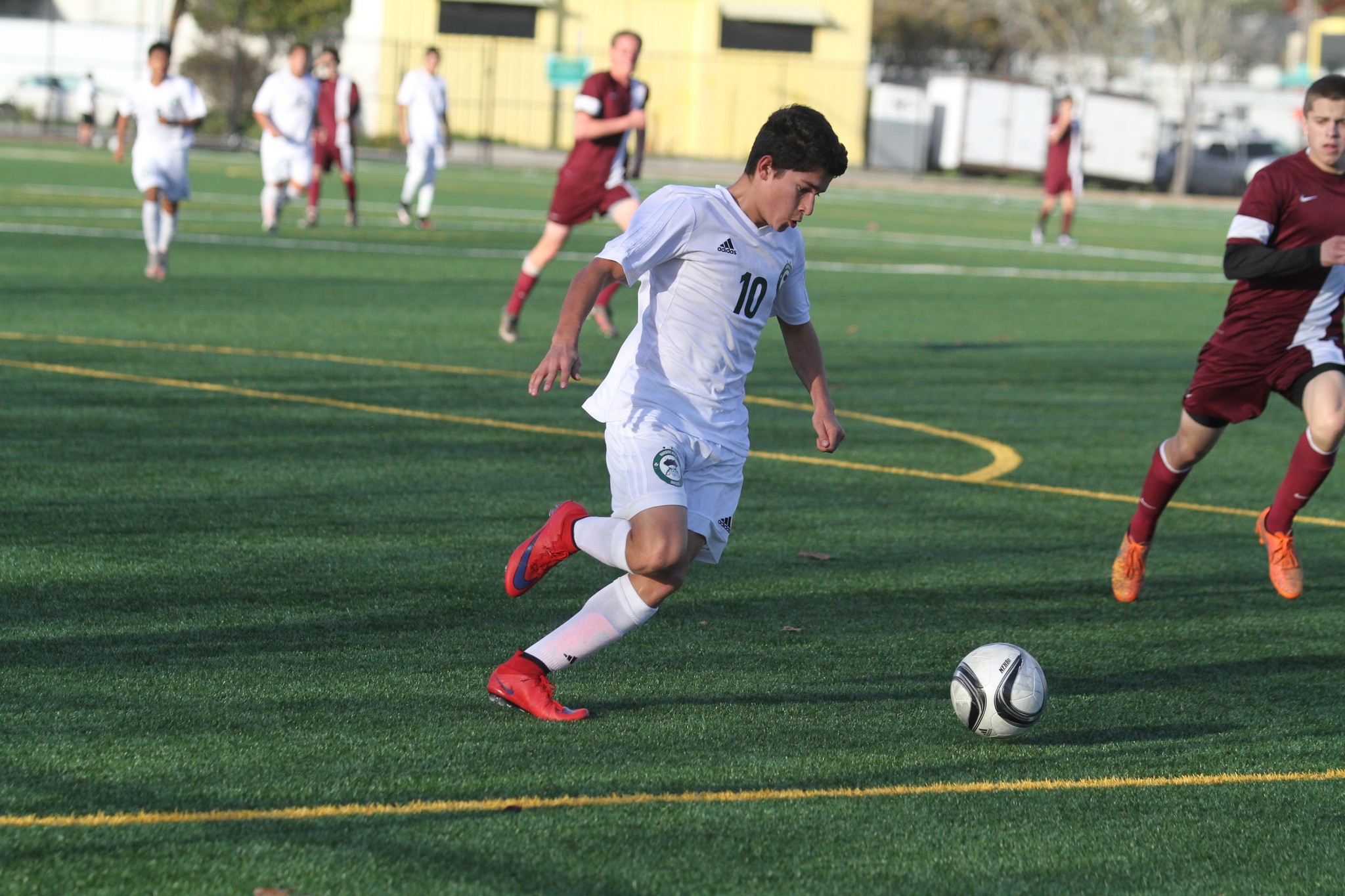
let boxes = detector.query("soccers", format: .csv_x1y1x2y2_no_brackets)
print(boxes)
949,641,1048,738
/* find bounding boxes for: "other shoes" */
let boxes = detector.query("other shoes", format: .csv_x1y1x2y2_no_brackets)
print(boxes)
346,213,355,228
145,248,166,282
260,217,278,232
398,204,412,225
500,309,518,344
418,218,432,230
305,204,318,227
590,305,619,339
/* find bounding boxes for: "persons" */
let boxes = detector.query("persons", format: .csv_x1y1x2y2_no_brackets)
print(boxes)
1032,95,1092,245
68,46,454,227
1109,70,1345,602
251,43,322,232
499,30,652,342
109,40,213,281
488,102,846,721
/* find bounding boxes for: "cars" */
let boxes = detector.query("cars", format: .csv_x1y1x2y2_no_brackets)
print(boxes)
1154,127,1289,199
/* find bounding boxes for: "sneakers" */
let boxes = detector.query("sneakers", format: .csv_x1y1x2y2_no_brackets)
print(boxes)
504,501,590,598
486,649,589,722
1109,523,1148,603
1255,505,1303,600
